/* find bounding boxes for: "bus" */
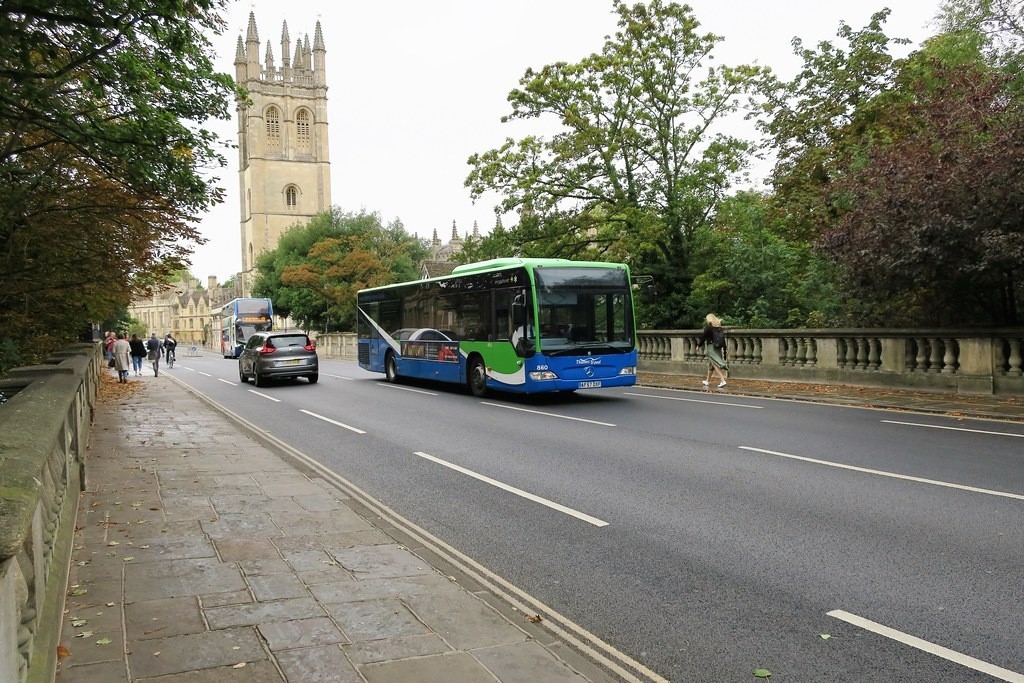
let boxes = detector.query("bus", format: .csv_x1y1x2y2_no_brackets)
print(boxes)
356,256,656,400
219,296,278,359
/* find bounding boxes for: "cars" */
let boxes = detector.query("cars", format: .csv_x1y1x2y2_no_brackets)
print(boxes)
238,330,319,387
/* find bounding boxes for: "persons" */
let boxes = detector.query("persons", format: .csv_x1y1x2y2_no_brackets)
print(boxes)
198,339,205,347
695,313,729,388
100,331,128,367
513,315,542,348
130,334,145,377
111,333,131,383
147,332,165,376
163,333,177,363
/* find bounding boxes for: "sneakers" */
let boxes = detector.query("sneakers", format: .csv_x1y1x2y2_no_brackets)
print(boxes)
717,381,727,388
702,380,710,386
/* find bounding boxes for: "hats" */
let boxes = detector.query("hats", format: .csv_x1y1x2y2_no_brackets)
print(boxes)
150,332,157,339
166,333,171,337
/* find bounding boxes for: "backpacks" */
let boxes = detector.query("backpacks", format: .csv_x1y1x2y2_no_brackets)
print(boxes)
706,325,726,348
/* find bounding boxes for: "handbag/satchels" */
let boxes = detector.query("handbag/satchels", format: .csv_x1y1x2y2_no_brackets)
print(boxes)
142,348,147,358
108,355,115,367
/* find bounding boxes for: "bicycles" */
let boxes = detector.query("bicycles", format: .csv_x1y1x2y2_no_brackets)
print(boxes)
164,346,175,369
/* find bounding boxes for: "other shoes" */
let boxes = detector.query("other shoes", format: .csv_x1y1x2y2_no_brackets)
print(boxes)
119,381,122,383
135,374,137,377
139,373,141,375
174,358,175,361
124,379,126,383
155,372,158,377
